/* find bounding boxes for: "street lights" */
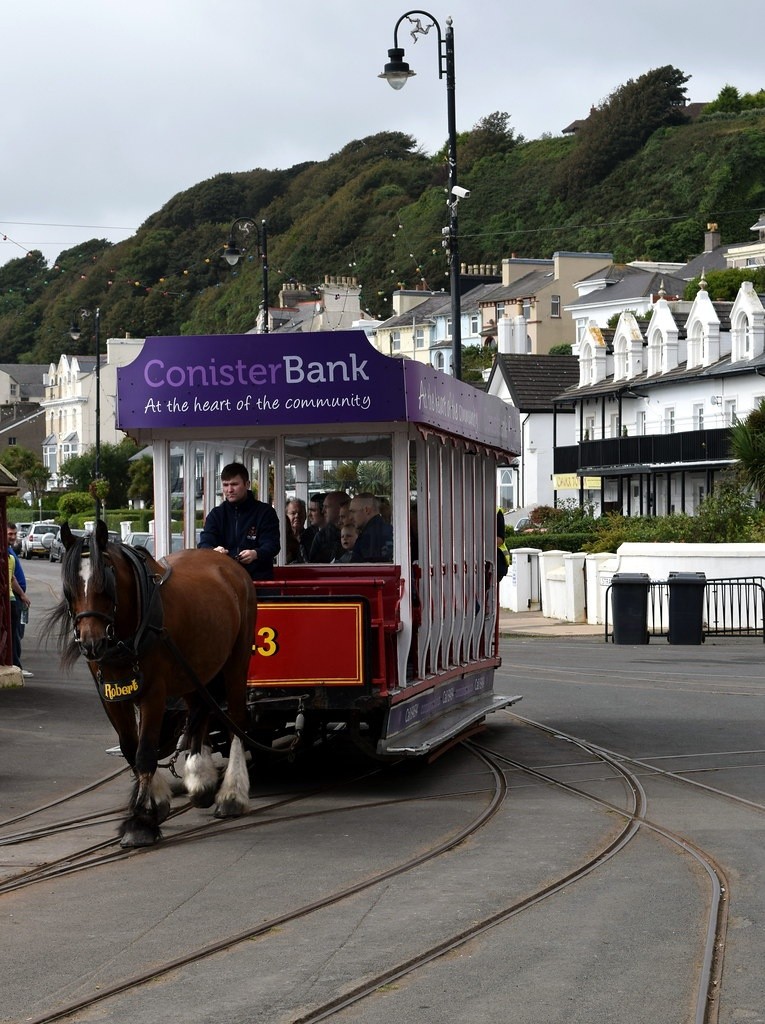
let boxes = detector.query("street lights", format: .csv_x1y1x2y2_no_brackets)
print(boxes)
378,7,463,381
68,303,101,520
220,216,268,333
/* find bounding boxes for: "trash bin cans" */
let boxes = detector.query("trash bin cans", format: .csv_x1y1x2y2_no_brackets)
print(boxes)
611,573,651,645
666,572,707,645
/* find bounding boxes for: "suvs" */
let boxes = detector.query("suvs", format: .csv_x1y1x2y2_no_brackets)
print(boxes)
20,524,60,560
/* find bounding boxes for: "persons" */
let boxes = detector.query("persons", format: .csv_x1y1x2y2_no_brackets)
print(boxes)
267,488,392,563
497,505,510,584
8,522,34,677
197,463,281,581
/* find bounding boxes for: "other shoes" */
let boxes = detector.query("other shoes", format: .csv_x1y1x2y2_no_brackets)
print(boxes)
22,670,34,678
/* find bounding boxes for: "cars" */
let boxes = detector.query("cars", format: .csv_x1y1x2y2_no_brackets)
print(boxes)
47,529,85,563
10,522,33,555
121,531,151,548
80,531,120,545
140,535,184,562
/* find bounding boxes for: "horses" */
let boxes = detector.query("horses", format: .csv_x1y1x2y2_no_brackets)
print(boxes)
32,520,258,848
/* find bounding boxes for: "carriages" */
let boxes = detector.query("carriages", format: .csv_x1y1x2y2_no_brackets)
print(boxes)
26,330,524,852
